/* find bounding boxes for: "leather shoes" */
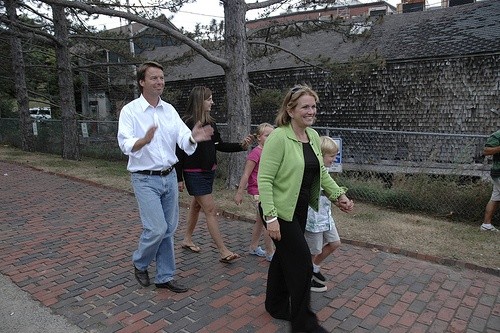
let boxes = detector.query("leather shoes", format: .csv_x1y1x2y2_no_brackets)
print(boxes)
159,280,188,292
134,265,150,286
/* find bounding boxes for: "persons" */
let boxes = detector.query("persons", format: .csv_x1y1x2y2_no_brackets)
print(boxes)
117,60,213,292
480,129,500,232
256,84,353,333
234,123,279,263
304,135,354,293
174,86,252,264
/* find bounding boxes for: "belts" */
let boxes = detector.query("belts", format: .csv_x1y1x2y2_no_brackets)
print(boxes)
131,164,174,175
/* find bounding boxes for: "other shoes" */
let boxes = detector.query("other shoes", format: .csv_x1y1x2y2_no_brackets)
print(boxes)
480,225,499,231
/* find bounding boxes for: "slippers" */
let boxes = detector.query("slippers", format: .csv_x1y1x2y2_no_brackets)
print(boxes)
181,242,200,252
219,253,242,263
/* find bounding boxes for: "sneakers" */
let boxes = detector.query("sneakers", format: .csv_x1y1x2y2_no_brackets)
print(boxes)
313,271,326,282
310,278,327,291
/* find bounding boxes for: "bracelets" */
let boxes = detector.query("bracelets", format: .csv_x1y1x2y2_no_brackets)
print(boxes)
266,217,278,223
189,135,196,145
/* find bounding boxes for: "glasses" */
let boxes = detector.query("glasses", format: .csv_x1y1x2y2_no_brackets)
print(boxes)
288,88,302,103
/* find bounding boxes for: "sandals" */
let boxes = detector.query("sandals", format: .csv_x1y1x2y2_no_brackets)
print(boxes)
249,246,266,256
265,251,274,261
